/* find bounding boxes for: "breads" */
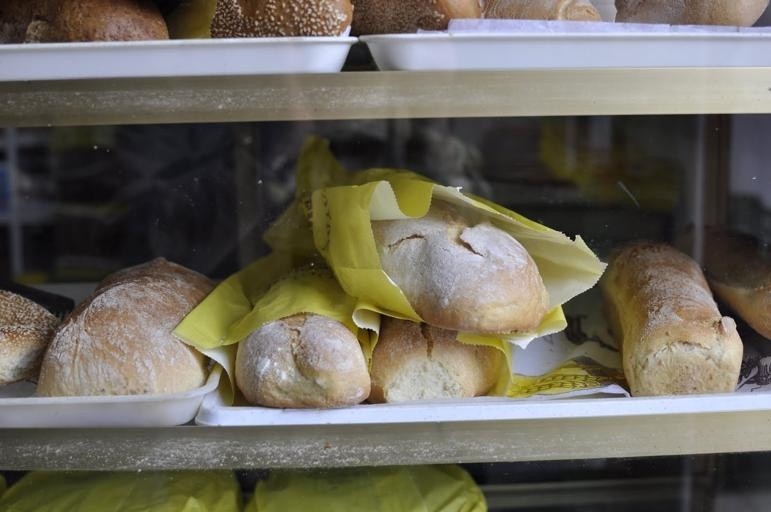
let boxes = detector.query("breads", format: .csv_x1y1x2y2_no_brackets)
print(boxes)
235,168,550,408
481,0,603,21
169,0,354,39
671,224,771,340
599,237,745,396
349,0,484,36
0,202,223,410
615,0,771,28
0,0,169,43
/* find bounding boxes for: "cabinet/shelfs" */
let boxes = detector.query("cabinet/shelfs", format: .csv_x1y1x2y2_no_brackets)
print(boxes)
0,66,770,472
